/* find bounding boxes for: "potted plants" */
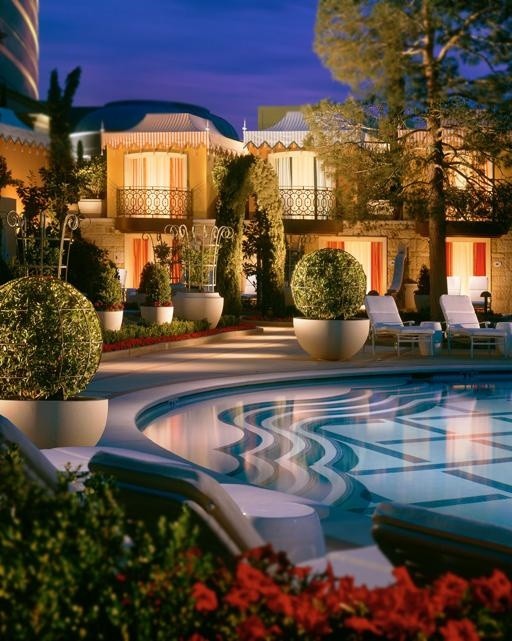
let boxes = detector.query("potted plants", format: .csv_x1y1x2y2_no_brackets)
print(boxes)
89,266,126,333
164,224,234,329
138,262,175,324
5,209,80,277
290,247,370,361
0,276,109,450
414,263,432,313
495,314,512,358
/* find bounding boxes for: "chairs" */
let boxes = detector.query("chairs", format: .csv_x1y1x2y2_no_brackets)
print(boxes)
439,294,508,365
0,415,72,492
371,500,510,589
87,451,267,561
364,294,434,358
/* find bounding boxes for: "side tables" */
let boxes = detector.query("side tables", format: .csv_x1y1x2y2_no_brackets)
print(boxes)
419,320,443,356
